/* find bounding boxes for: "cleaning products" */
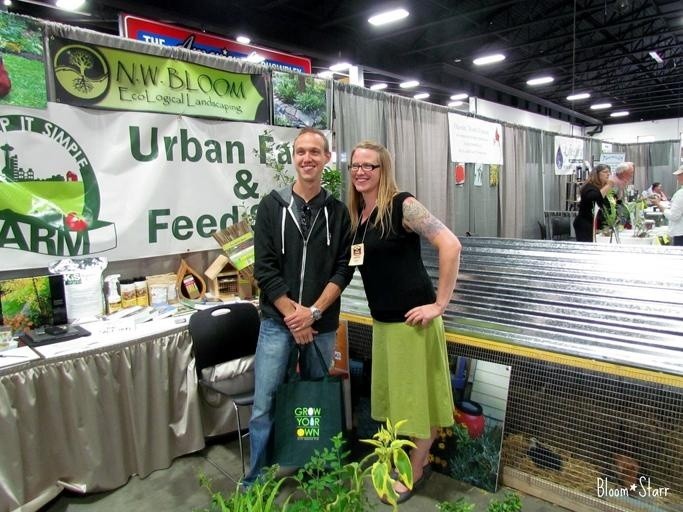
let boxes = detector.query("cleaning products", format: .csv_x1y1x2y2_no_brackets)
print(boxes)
105,274,122,314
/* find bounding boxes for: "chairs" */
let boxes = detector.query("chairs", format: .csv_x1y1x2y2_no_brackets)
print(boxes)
189,303,260,486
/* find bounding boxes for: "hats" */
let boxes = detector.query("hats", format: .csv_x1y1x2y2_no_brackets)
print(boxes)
673,165,683,175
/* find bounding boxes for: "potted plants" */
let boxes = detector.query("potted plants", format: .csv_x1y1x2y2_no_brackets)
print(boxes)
595,191,655,245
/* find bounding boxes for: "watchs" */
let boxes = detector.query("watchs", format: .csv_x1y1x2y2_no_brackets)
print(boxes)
308,304,322,321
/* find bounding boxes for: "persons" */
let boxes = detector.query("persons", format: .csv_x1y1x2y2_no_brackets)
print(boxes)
345,140,464,503
236,126,354,493
572,161,682,248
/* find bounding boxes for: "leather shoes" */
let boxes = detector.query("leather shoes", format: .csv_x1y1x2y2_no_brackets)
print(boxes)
380,460,431,505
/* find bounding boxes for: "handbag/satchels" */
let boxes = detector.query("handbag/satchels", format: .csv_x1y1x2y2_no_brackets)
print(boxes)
267,369,342,469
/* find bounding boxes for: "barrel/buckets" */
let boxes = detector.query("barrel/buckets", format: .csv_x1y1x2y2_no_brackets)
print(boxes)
456,399,484,436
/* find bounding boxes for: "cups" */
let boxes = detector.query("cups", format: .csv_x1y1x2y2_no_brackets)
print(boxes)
0,325,13,351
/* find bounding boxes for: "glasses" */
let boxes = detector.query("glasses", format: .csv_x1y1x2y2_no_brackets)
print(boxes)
348,163,380,172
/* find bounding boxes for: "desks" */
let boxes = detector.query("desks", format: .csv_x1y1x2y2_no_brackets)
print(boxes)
0,308,255,512
